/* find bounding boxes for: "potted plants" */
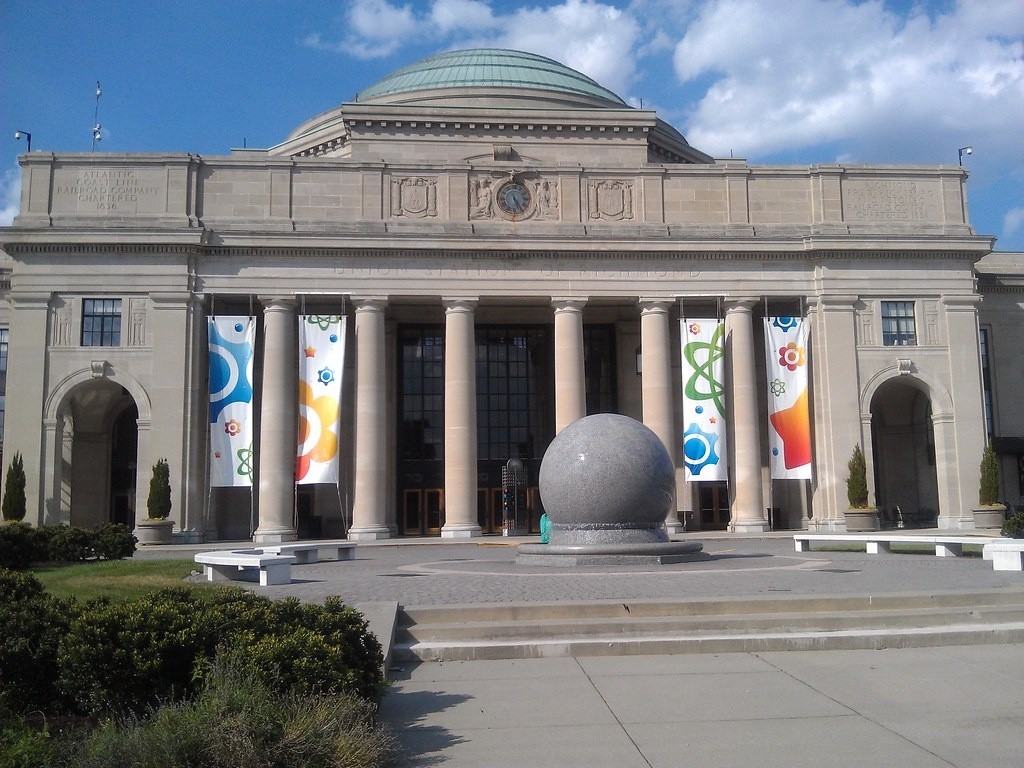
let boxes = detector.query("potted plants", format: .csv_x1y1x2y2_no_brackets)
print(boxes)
971,438,1007,529
138,457,175,545
843,442,880,532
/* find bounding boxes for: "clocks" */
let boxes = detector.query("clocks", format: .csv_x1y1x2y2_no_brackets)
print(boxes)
498,184,531,214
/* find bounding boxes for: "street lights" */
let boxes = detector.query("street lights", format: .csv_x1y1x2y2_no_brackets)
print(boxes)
15,130,31,152
959,146,973,166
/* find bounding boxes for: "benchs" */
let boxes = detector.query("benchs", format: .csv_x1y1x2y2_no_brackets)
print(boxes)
985,539,1024,572
255,542,358,565
194,549,297,586
794,534,1014,561
